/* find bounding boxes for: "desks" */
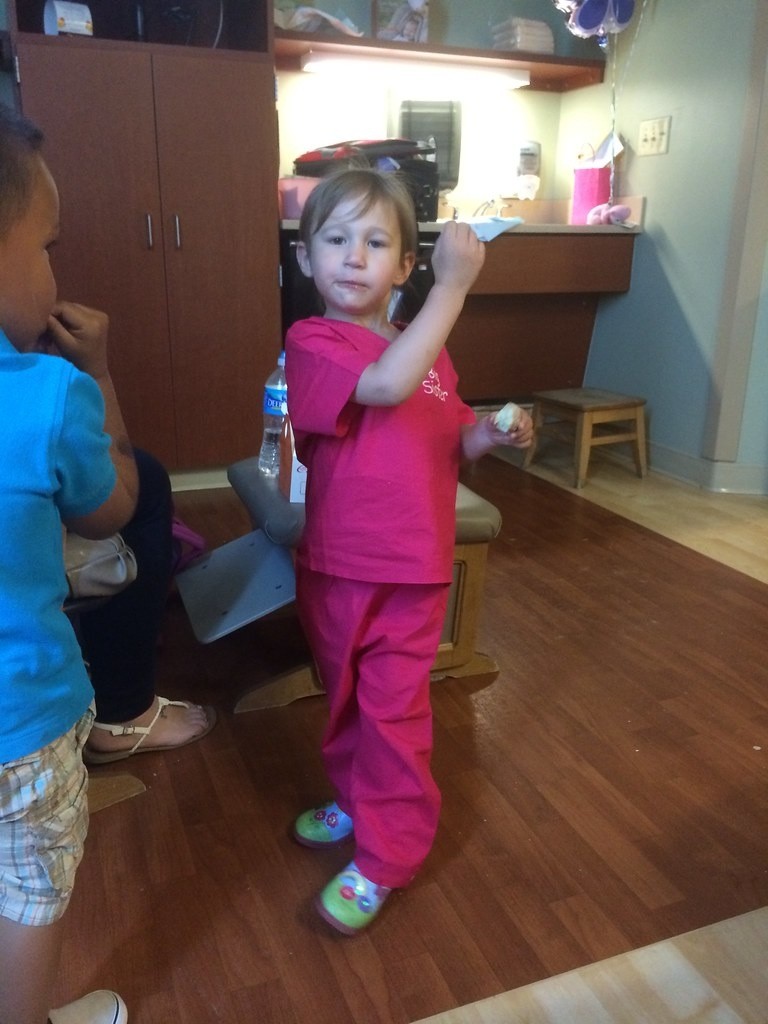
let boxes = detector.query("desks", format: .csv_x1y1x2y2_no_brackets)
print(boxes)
227,457,503,715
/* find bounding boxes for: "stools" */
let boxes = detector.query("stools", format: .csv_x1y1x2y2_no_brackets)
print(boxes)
518,387,648,490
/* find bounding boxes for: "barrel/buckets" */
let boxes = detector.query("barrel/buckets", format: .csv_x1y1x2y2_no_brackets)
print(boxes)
572,168,610,225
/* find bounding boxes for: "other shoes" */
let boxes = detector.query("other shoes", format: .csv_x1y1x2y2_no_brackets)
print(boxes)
45,989,129,1024
294,799,356,851
314,860,391,937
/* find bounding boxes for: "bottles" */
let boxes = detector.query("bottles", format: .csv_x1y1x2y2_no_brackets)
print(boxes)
258,351,288,477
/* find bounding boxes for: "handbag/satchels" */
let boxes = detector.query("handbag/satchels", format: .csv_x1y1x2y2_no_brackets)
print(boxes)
60,519,139,601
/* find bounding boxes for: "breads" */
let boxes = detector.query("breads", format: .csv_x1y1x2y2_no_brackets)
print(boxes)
496,402,522,434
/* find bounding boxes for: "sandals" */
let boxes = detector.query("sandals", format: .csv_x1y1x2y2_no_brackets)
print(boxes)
82,694,216,763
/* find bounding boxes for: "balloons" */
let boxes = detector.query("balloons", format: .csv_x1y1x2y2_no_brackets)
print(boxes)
555,0,637,49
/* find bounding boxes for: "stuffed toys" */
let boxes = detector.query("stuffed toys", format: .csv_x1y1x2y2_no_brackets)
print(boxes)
587,203,631,226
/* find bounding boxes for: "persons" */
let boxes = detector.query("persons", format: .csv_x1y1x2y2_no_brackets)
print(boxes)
0,110,215,1023
284,167,534,935
394,18,418,41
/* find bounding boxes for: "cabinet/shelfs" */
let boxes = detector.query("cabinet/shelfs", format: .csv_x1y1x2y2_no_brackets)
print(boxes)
6,0,283,474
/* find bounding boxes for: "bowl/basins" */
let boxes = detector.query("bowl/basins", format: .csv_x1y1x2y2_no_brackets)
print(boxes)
279,178,321,220
